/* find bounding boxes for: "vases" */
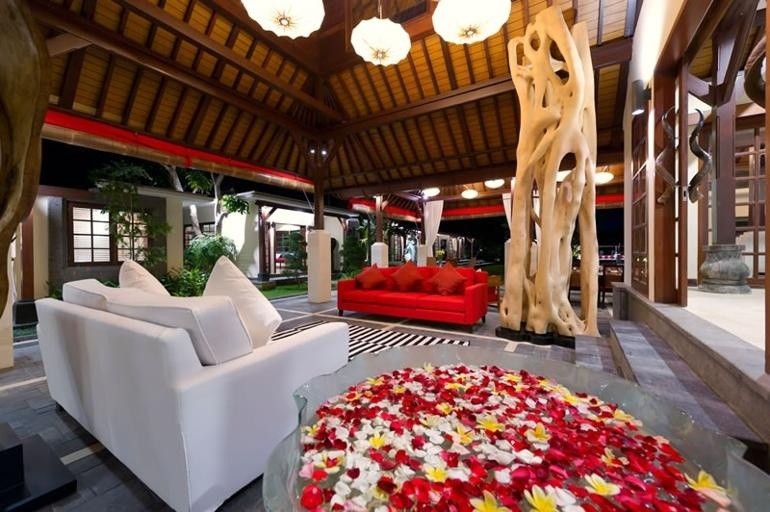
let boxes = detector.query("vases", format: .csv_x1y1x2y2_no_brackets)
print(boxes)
298,362,732,512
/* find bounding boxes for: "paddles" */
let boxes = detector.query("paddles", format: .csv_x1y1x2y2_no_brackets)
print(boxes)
274,318,472,363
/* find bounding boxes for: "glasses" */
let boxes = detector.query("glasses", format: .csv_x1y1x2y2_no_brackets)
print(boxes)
427,0,511,45
350,1,412,70
241,0,325,39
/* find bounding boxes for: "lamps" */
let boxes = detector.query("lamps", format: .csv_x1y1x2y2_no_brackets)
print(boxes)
598,263,627,309
567,264,581,302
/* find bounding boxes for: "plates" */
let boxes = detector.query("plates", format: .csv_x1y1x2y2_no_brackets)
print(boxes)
488,274,501,313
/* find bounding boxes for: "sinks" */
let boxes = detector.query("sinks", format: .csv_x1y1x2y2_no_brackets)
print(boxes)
33,260,348,512
337,261,491,331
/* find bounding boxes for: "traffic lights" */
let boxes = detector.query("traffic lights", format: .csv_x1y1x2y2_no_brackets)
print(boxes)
426,260,467,297
354,262,387,291
199,257,279,348
117,261,171,295
387,261,425,294
